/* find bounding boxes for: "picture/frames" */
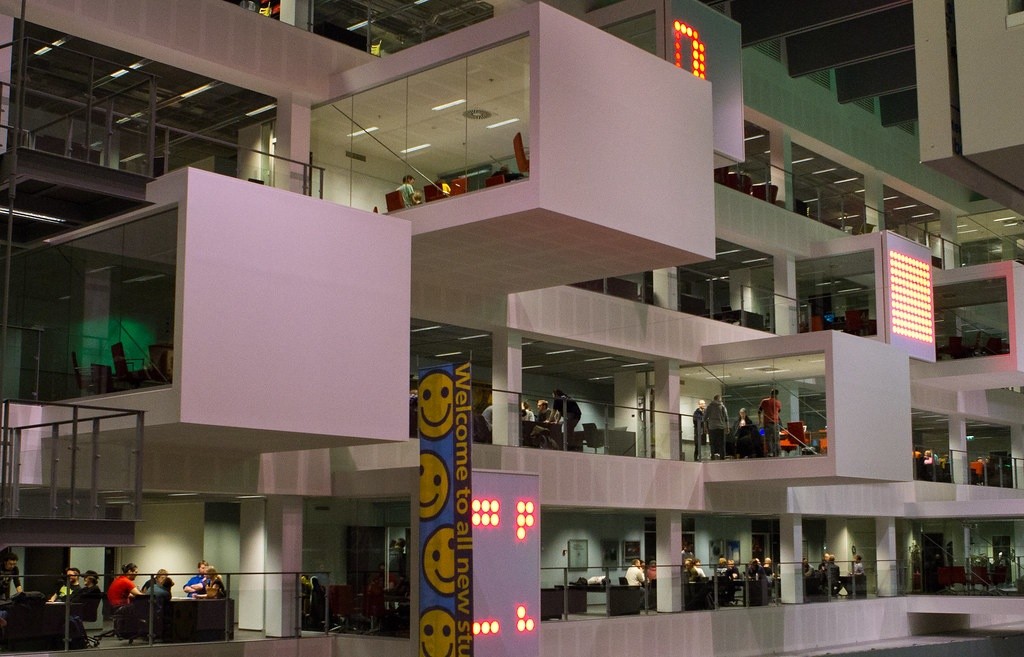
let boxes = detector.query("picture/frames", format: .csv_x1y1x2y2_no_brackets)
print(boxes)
568,539,589,572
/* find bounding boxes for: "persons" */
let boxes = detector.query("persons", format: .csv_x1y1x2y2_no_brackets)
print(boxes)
913,447,995,486
396,175,422,208
847,555,864,576
301,537,406,622
107,560,226,615
0,553,22,626
693,388,783,461
482,389,582,451
436,179,451,193
48,568,101,619
993,548,1008,587
624,541,843,609
492,165,524,182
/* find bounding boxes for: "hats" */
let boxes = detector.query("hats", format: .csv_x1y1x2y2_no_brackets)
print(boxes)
79,571,98,581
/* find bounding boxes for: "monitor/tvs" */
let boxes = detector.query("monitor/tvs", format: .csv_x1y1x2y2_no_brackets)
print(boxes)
824,313,835,322
759,427,765,435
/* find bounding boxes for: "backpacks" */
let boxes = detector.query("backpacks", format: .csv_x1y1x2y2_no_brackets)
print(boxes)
11,591,47,605
539,434,559,450
704,592,714,610
70,616,88,650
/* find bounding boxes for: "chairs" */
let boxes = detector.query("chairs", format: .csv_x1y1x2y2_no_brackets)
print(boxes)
736,425,764,459
793,197,807,217
71,351,98,397
713,166,778,204
384,132,529,213
110,594,164,646
845,310,865,336
583,423,628,455
786,420,819,455
693,575,734,607
576,577,629,585
473,413,492,444
325,584,386,636
912,572,922,591
811,314,825,331
939,565,1007,597
916,456,984,486
947,331,1003,360
112,341,145,390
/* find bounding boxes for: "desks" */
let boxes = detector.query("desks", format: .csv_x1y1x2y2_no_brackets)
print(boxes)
540,583,642,621
803,574,867,600
567,277,637,303
170,597,235,643
570,429,604,451
645,283,705,316
42,601,84,650
522,420,562,450
734,578,770,607
700,310,764,333
687,582,715,609
